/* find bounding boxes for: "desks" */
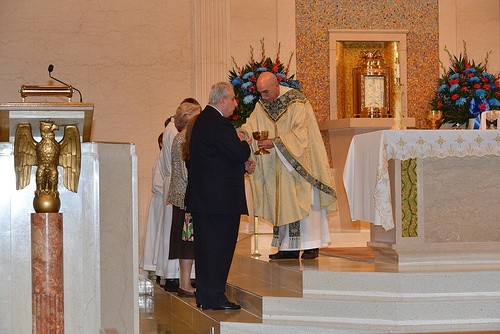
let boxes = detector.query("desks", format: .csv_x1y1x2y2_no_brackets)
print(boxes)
341,130,500,251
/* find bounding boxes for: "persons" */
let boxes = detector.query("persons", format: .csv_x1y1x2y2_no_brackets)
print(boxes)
139,82,256,310
238,71,338,261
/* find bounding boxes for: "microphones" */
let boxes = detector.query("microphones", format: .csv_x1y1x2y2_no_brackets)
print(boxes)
48,65,82,102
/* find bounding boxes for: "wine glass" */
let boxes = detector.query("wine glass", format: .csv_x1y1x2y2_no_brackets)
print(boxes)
423,110,443,129
486,111,497,129
252,131,272,155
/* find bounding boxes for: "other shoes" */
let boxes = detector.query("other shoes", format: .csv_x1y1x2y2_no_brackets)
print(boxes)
301,249,319,259
197,301,241,310
164,280,195,291
175,287,197,297
269,251,299,259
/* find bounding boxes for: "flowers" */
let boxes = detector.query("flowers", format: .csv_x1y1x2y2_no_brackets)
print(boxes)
427,38,500,130
228,37,304,129
